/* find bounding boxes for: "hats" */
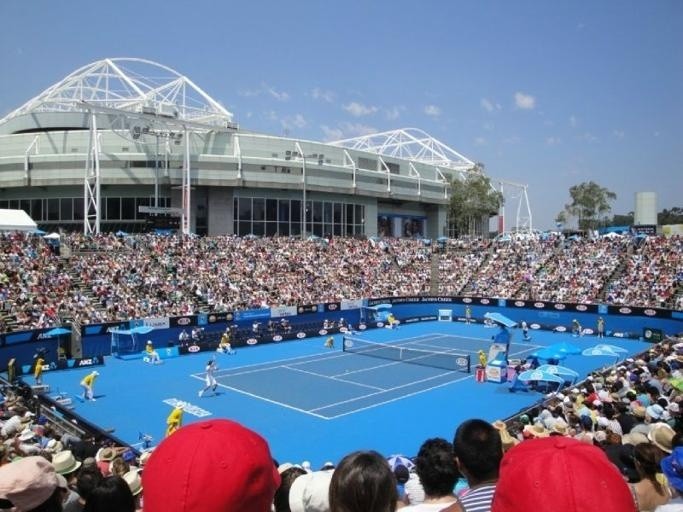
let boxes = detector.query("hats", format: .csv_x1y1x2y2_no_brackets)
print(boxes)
608,393,683,492
635,359,646,366
493,403,576,437
676,356,683,363
492,437,638,512
288,469,336,512
142,419,280,512
0,427,151,512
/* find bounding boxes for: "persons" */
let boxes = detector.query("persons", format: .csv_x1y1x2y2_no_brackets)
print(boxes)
1,229,683,512
